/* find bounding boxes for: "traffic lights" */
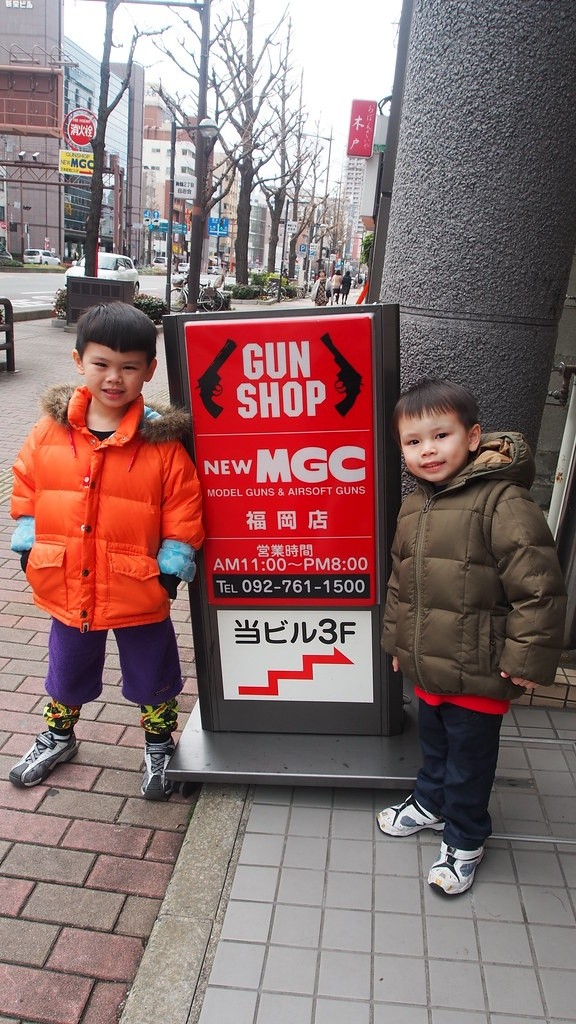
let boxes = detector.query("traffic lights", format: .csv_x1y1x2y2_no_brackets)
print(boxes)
220,219,224,230
23,206,31,210
148,225,159,229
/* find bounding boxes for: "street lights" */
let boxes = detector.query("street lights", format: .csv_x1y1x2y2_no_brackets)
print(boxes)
319,174,342,247
301,127,332,270
166,118,218,315
217,212,232,266
125,166,150,257
327,240,342,278
277,199,310,302
305,224,328,281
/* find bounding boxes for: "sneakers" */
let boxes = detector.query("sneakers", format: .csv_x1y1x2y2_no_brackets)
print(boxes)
8,731,77,787
425,835,484,895
139,738,177,801
376,795,448,838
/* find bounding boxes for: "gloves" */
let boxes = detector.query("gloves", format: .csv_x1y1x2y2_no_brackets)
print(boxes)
158,571,182,600
19,547,33,573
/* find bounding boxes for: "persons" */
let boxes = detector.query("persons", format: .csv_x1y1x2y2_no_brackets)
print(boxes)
353,276,362,289
311,269,351,306
283,268,288,285
73,249,80,262
10,300,204,811
377,378,568,902
134,257,139,268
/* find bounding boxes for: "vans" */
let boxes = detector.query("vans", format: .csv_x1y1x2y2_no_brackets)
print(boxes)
23,249,61,266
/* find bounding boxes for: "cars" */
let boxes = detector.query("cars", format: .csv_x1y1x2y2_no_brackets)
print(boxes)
0,242,13,260
64,252,141,295
153,257,190,274
208,266,221,275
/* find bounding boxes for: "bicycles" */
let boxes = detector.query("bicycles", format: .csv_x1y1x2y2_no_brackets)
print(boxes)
170,274,224,312
258,282,287,302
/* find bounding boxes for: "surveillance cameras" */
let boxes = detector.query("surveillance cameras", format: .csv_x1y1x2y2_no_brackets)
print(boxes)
18,151,26,160
32,152,40,160
